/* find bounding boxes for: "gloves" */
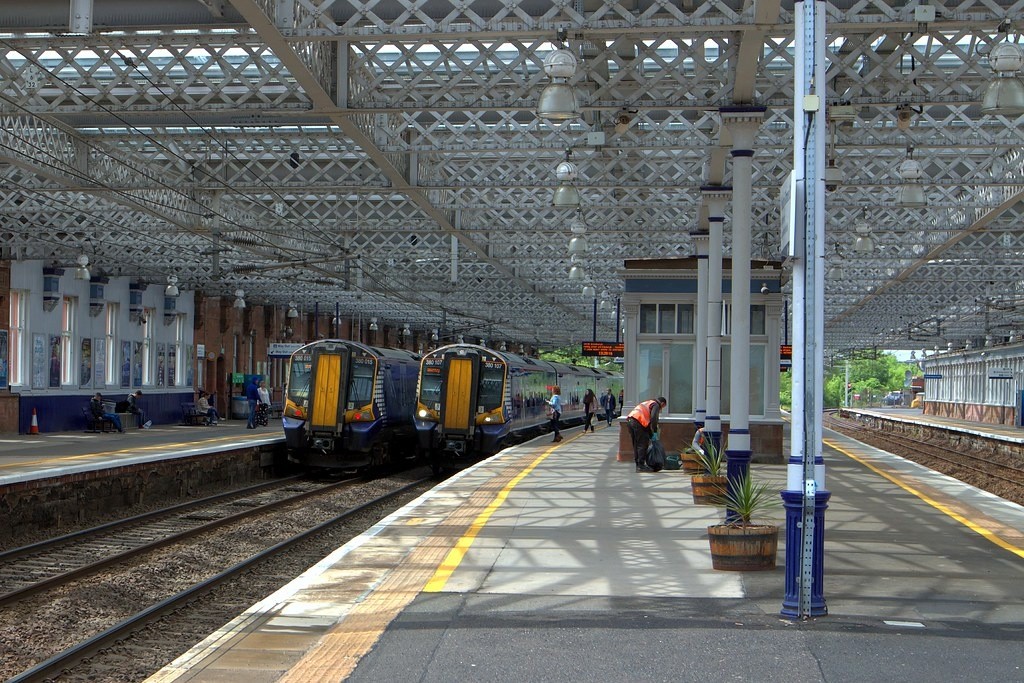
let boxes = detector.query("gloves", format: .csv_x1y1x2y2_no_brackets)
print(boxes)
651,432,657,442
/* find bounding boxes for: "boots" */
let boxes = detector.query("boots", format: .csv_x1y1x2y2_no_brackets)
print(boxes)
552,433,562,442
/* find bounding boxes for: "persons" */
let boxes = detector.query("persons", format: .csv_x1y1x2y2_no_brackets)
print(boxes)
90,392,127,433
126,390,146,429
583,389,598,434
687,428,706,455
602,388,624,427
626,397,667,472
245,377,272,429
196,393,226,425
544,386,564,442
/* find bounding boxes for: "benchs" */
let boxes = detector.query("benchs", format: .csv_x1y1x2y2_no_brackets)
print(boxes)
81,406,117,434
271,402,283,420
181,402,213,426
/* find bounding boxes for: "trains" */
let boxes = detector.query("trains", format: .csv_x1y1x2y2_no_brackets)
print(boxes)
411,343,626,479
280,339,429,477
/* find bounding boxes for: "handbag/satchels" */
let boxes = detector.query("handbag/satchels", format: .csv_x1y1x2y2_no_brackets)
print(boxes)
611,410,618,419
259,402,268,411
590,414,599,426
143,420,152,429
545,405,561,421
255,405,259,412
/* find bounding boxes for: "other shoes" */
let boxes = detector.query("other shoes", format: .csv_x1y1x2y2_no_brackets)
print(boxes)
581,430,586,433
219,417,226,421
118,428,126,433
138,425,143,429
636,466,654,473
209,423,215,426
590,431,594,433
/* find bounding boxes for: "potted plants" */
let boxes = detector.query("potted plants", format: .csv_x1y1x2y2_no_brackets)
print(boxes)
674,428,729,506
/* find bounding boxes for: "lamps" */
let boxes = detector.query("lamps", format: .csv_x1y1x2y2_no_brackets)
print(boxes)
403,323,525,353
332,310,342,324
553,148,578,207
568,210,623,331
164,270,182,297
826,243,843,280
288,299,298,318
899,144,926,207
910,330,1017,359
537,28,579,120
73,247,91,280
856,206,873,251
370,316,379,330
233,286,246,307
979,18,1024,115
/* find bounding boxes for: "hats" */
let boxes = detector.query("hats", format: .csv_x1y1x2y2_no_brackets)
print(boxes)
96,392,102,397
135,389,144,395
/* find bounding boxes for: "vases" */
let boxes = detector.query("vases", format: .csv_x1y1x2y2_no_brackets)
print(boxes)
680,452,708,474
699,463,785,570
